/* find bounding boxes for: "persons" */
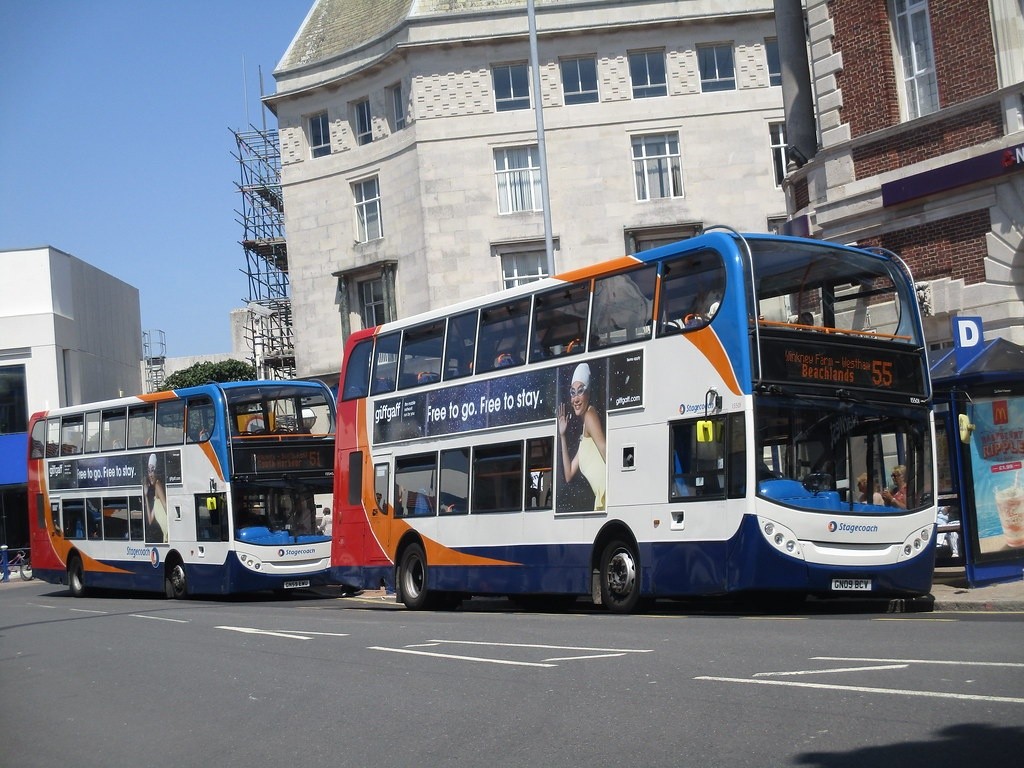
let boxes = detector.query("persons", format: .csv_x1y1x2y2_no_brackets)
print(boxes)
881,464,907,510
556,363,606,511
857,473,884,506
140,454,168,543
706,299,720,321
942,506,961,558
317,507,332,536
797,311,817,332
937,507,948,547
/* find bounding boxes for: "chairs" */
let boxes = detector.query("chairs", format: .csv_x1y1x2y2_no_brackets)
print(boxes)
111,427,209,447
345,312,712,400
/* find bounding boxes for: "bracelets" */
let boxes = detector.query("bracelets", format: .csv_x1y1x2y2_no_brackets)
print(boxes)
891,498,895,502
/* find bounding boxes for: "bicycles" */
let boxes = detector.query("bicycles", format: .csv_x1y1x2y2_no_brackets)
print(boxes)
0,551,32,581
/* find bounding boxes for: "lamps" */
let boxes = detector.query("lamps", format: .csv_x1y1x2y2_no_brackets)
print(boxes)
787,145,808,168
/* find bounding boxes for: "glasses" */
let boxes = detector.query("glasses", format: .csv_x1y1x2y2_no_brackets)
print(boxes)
891,474,903,478
146,467,156,474
570,385,588,397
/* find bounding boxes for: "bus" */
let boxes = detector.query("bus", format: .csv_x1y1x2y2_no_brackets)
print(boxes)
331,224,975,611
27,378,337,600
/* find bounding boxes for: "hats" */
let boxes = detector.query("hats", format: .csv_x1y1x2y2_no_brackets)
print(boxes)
571,363,591,387
147,454,157,467
